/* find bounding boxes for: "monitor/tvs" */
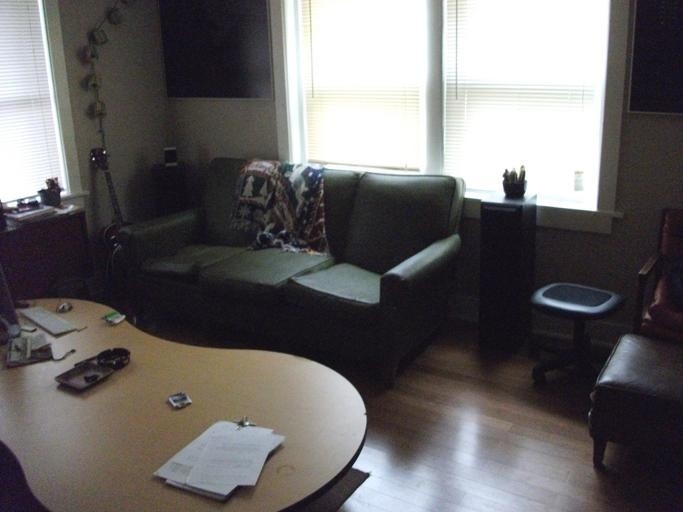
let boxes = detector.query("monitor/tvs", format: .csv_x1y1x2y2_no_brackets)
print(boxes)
0,262,21,344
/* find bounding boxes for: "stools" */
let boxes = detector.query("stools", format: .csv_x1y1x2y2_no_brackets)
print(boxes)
527,283,626,383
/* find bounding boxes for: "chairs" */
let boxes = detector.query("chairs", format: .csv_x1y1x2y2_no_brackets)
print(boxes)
587,207,683,470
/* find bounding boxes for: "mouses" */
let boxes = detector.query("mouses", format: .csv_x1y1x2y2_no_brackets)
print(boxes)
56,302,73,312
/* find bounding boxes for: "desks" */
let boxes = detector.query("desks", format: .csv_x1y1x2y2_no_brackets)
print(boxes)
0,297,370,511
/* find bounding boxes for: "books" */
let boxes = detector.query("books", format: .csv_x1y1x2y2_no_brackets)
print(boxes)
3,204,54,222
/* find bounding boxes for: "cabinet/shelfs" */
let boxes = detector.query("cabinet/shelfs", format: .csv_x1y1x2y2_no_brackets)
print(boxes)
0,202,93,309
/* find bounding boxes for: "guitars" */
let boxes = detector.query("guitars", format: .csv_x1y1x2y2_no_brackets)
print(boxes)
91,146,132,274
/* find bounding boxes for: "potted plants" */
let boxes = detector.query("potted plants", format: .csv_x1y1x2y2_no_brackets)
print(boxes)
502,164,528,199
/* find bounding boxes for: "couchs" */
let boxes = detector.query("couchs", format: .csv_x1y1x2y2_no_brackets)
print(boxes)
114,156,468,391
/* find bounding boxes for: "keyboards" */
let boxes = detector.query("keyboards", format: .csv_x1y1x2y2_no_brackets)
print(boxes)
20,307,77,335
